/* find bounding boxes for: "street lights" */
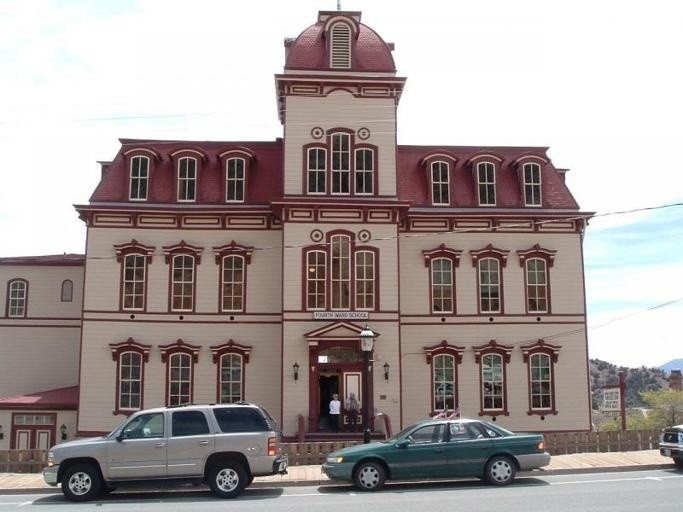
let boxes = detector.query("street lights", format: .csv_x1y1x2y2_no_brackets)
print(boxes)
358,324,377,444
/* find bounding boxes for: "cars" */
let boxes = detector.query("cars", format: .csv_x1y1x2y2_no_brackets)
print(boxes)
321,417,551,491
42,400,289,502
659,424,683,468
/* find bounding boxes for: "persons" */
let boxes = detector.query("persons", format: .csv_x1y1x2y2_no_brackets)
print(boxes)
345,392,360,432
328,394,341,431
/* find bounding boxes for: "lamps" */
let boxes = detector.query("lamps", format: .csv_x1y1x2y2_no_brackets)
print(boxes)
0,425,3,440
383,362,389,380
61,424,67,440
292,361,299,382
